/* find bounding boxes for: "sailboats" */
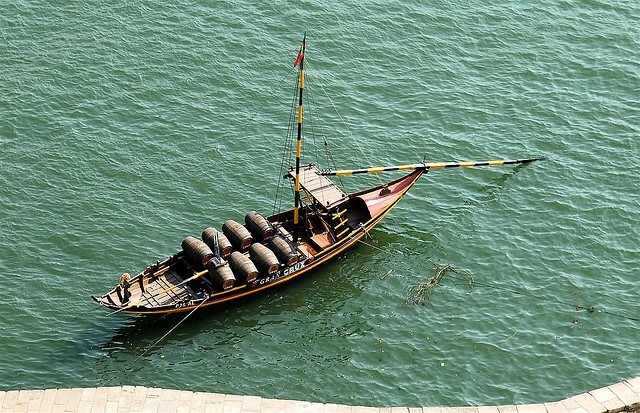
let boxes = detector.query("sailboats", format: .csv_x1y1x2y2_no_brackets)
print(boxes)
91,30,548,317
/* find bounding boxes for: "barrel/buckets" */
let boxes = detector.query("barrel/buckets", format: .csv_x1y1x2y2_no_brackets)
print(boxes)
244,211,275,240
202,227,232,257
182,235,214,266
222,220,253,250
249,242,280,274
207,257,236,290
228,251,259,283
269,235,299,267
